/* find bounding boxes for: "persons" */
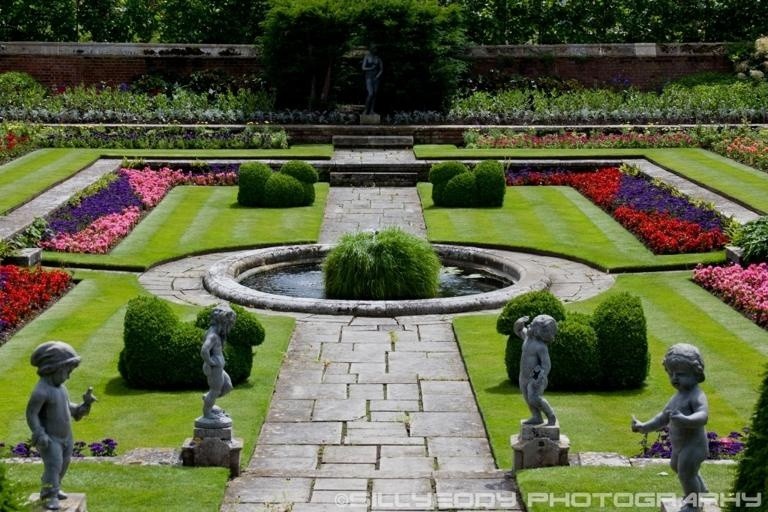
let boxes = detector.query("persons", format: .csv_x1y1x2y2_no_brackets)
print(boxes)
23,338,100,511
629,341,711,511
201,303,235,420
362,44,384,115
512,312,559,425
